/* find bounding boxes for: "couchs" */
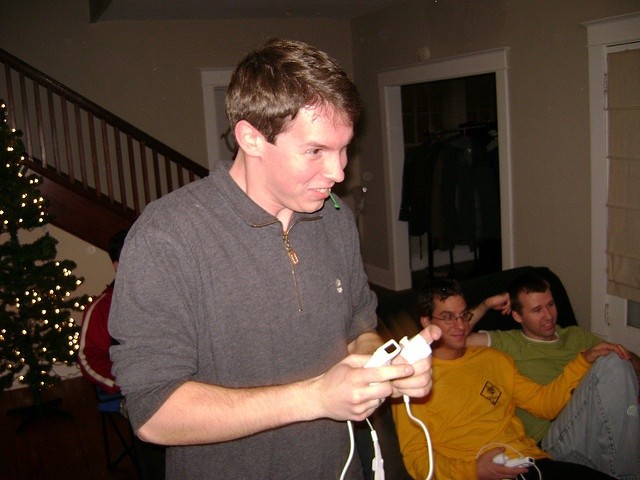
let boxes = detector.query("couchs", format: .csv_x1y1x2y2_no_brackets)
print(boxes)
134,265,578,479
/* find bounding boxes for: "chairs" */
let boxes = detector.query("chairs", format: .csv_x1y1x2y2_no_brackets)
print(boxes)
91,381,137,471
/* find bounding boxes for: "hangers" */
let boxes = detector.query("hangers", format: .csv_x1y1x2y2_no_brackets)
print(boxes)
425,124,477,156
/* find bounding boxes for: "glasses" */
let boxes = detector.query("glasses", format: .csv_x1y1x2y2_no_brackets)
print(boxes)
432,310,473,322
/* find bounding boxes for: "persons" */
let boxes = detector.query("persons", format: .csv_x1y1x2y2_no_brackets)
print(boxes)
464,276,640,480
109,38,442,479
77,231,166,479
391,276,631,480
